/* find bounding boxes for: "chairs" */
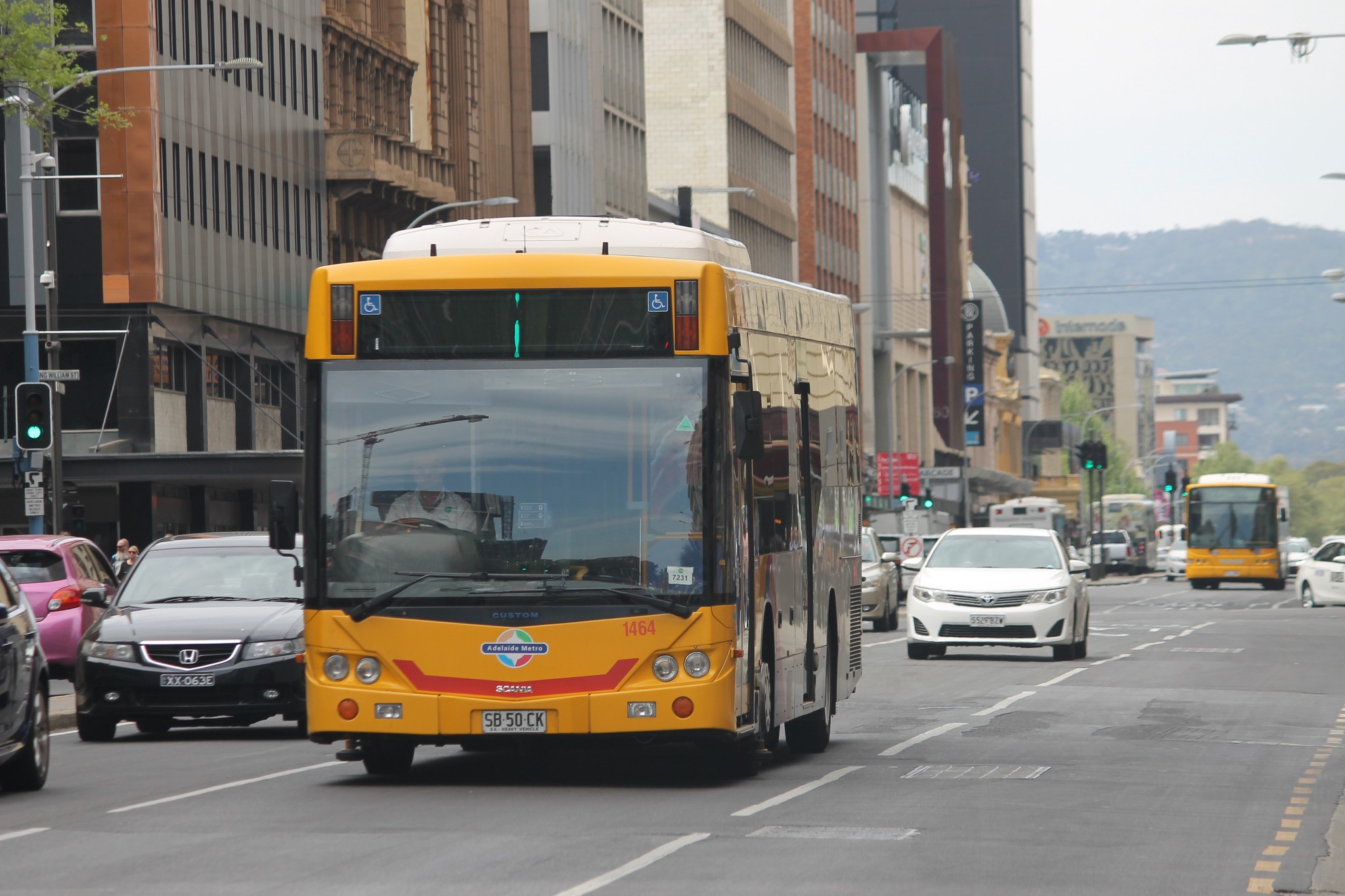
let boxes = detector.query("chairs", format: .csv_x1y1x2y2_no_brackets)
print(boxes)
240,573,276,600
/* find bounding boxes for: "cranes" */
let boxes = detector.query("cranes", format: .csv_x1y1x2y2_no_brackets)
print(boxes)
325,413,490,521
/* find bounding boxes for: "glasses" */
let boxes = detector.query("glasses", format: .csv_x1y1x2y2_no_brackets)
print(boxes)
116,545,126,548
128,551,136,554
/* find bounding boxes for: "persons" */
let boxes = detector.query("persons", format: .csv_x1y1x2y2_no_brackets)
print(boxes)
373,447,479,537
110,538,139,581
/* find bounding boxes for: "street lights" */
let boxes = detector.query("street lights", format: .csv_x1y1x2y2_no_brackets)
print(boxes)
18,56,265,534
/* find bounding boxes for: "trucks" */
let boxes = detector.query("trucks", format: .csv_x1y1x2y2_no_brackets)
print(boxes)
988,495,1068,546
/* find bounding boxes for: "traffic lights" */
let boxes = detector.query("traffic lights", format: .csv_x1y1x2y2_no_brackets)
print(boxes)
1180,477,1191,498
1094,444,1108,472
1075,441,1095,470
1164,470,1177,495
14,381,55,452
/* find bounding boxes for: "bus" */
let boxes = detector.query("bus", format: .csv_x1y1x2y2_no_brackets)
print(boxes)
265,213,868,780
1099,492,1157,572
1183,472,1288,591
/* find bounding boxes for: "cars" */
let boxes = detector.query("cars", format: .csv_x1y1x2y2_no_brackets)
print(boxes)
0,557,54,793
0,533,120,681
71,528,312,744
898,526,1091,662
1082,523,1345,582
861,526,941,632
1294,539,1345,608
